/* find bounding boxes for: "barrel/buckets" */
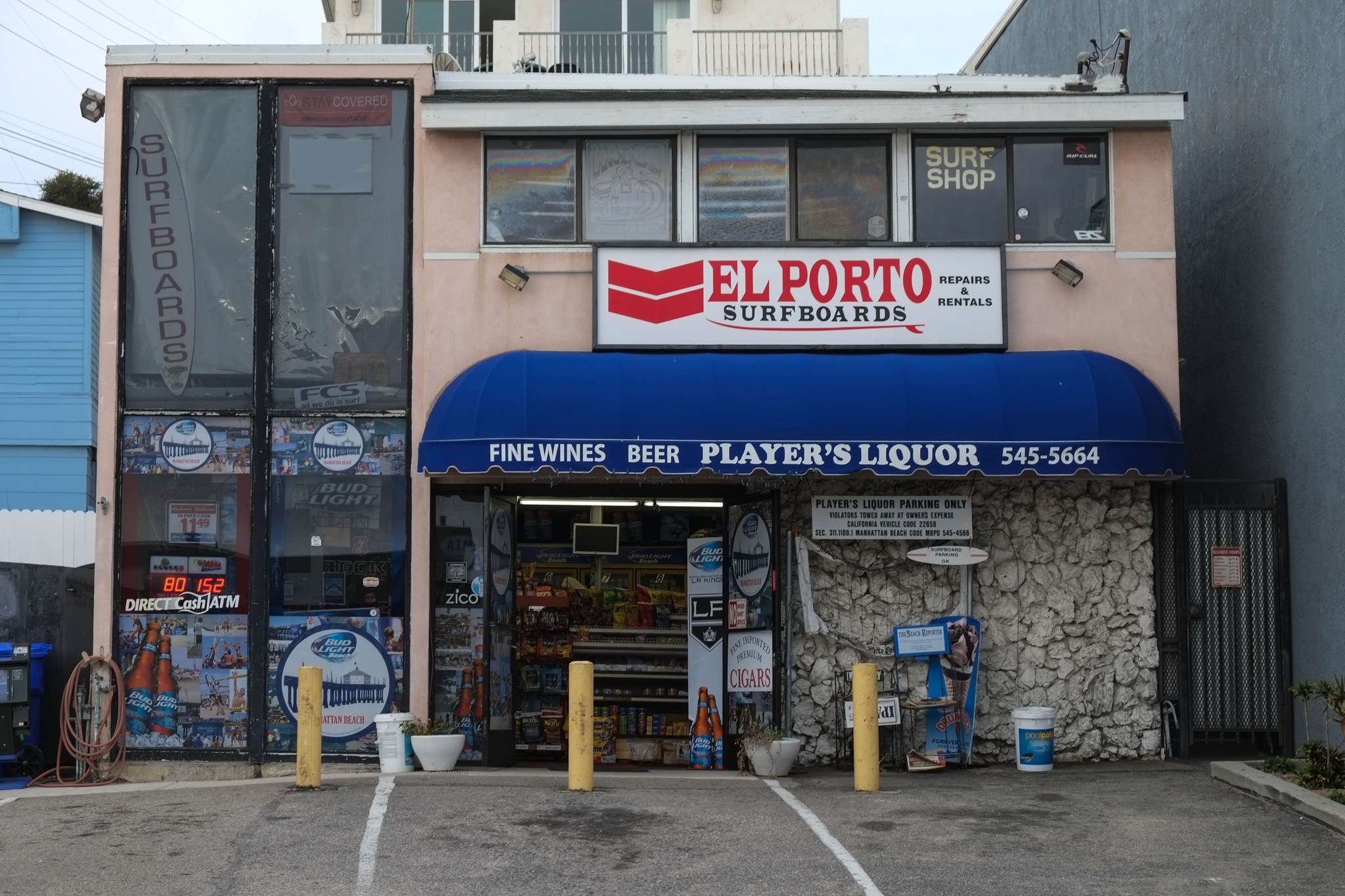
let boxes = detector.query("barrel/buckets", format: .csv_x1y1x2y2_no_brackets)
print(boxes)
373,712,415,773
1010,707,1057,772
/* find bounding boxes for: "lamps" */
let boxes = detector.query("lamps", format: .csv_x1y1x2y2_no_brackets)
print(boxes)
497,261,530,292
78,85,105,123
515,494,725,512
1051,257,1085,289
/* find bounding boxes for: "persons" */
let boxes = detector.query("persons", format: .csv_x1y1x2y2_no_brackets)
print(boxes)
119,615,402,747
435,618,471,730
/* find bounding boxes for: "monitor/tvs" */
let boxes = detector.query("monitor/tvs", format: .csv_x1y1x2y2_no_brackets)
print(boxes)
572,522,620,555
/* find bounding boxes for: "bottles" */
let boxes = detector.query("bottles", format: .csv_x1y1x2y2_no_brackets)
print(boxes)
148,635,179,738
454,669,473,759
473,657,483,752
120,621,161,735
689,686,712,769
708,694,723,769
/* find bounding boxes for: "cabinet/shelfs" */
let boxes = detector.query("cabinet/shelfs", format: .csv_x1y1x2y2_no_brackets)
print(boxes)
512,564,691,770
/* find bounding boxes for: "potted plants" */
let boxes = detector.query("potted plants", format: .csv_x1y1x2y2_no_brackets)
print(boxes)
737,708,802,779
402,700,467,774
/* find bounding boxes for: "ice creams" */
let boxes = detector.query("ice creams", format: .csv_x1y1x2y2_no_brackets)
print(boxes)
939,616,978,733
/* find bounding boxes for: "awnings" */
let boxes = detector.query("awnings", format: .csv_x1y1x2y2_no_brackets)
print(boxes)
417,350,1188,480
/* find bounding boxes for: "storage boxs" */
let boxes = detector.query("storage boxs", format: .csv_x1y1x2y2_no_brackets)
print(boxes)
663,738,686,751
616,737,662,760
664,750,689,764
673,721,689,735
